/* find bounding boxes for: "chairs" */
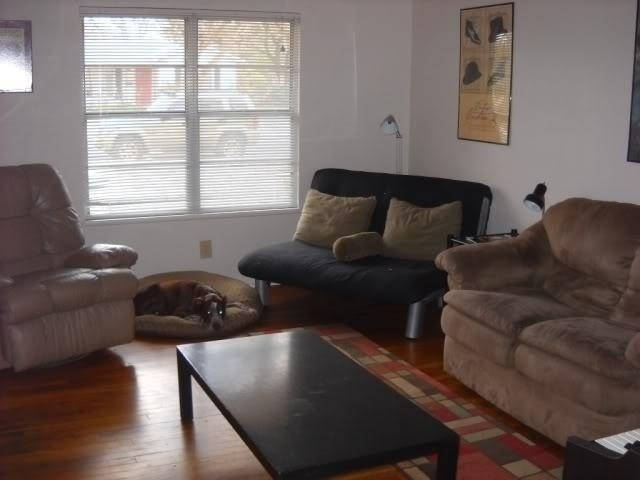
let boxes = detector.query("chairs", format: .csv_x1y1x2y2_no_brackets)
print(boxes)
0,162,141,374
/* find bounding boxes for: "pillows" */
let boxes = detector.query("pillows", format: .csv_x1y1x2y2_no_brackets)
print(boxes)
292,188,464,263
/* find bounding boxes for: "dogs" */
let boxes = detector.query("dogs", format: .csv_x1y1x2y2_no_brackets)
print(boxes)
132,278,241,331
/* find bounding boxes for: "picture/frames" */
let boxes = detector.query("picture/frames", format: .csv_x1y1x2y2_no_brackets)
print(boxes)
0,19,34,94
455,1,514,147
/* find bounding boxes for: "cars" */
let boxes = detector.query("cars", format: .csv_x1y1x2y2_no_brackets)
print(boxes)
94,89,259,159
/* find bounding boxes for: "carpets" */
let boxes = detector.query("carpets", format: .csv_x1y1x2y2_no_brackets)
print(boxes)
246,321,564,480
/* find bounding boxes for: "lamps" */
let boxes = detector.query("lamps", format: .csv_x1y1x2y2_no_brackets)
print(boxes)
522,181,548,222
379,114,406,174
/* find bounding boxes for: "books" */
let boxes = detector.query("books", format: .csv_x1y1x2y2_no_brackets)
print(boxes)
466,232,514,246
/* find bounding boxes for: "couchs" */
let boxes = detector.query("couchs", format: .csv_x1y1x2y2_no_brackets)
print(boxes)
432,197,640,448
237,167,495,342
